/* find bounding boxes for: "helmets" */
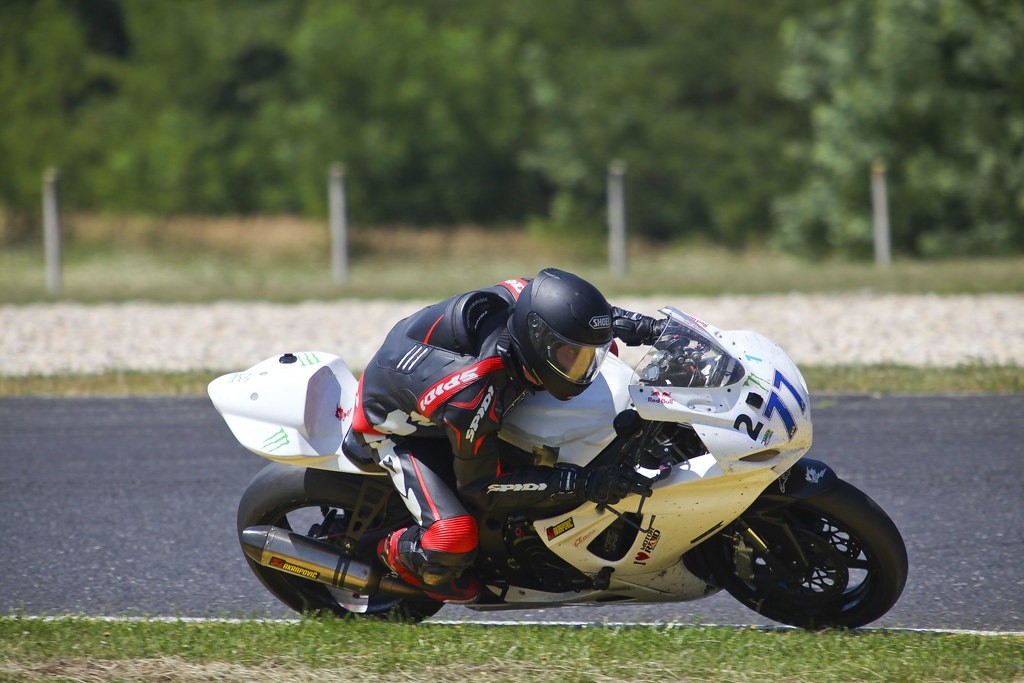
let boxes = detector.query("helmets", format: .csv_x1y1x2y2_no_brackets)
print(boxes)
508,267,615,401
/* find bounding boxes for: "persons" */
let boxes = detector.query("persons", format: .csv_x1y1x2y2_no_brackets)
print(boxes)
352,268,710,604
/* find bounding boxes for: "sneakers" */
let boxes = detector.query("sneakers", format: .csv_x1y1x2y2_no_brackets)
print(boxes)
378,527,480,605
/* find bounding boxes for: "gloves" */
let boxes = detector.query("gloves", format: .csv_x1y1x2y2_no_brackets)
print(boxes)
548,457,628,505
611,304,692,355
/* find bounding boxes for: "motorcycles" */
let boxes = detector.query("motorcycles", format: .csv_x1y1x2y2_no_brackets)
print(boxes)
206,306,909,634
